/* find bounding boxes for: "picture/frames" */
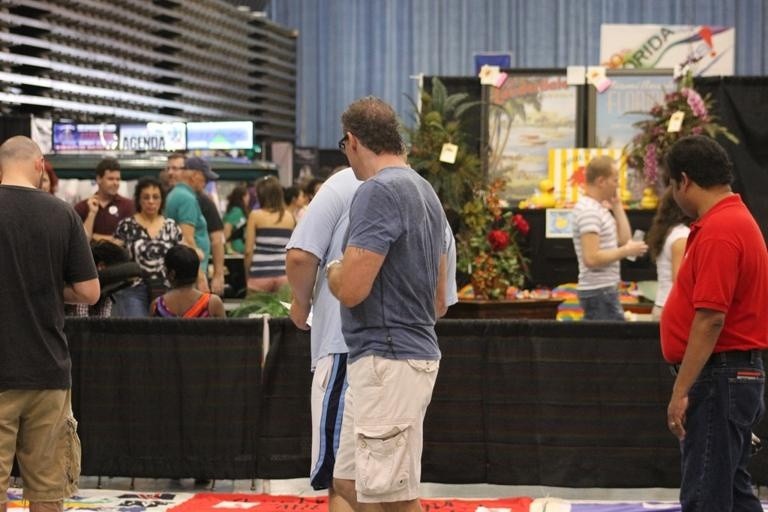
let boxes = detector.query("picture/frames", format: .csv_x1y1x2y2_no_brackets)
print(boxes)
586,64,683,219
478,67,585,207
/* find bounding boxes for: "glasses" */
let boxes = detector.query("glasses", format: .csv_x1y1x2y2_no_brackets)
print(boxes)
337,136,349,150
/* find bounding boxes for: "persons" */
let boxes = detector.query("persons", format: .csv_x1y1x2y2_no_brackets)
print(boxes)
570,153,648,322
324,96,448,512
40,153,348,318
0,135,100,512
285,142,458,512
646,185,692,321
660,135,768,512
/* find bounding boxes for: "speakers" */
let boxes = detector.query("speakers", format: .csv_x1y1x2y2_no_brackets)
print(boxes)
208,254,247,299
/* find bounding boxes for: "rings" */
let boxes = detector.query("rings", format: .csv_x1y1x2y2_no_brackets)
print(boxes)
671,422,675,426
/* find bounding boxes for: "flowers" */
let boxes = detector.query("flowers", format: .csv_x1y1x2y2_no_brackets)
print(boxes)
453,180,533,298
617,68,740,198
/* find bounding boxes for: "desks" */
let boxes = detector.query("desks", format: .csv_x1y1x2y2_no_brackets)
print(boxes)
441,298,566,319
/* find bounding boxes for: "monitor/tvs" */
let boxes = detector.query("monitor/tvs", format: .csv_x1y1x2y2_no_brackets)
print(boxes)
51,119,254,152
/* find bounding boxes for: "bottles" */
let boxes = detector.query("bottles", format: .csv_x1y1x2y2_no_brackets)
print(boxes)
626,228,645,262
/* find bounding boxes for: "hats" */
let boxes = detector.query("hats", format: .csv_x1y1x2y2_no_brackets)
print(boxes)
184,157,219,180
